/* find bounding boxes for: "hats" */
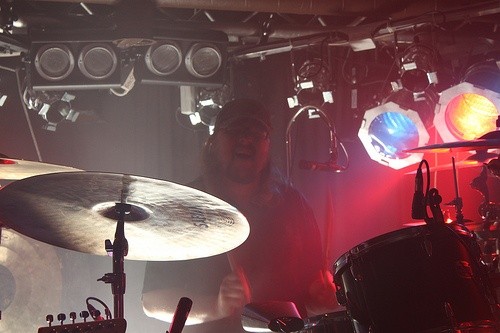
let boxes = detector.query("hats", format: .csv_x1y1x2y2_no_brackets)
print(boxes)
215,98,272,136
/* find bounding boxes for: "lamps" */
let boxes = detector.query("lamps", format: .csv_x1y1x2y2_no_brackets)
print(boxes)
290,23,500,169
189,87,230,134
23,86,80,132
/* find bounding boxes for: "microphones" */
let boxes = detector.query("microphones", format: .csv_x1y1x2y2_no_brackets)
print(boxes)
411,165,424,220
299,160,345,171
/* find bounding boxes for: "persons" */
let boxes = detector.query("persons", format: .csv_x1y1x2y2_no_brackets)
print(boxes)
141,98,343,325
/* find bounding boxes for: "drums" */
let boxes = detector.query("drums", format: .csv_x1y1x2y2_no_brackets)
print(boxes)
332,223,489,332
306,309,356,333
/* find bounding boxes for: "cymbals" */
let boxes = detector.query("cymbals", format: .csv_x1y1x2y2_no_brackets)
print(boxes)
1,170,251,261
402,139,500,152
0,157,83,180
